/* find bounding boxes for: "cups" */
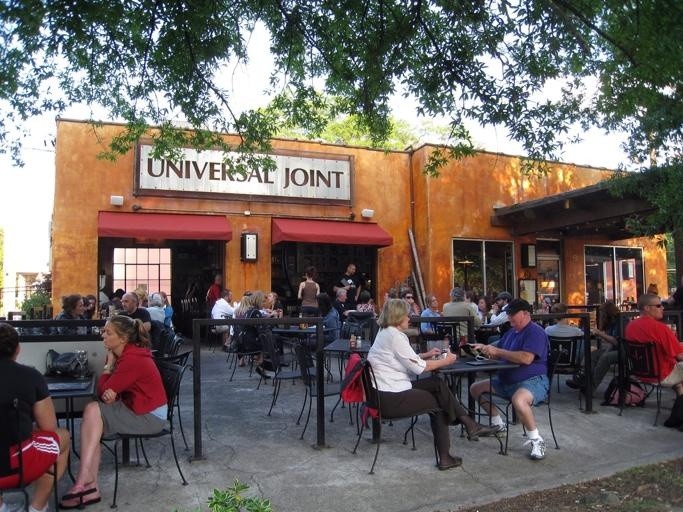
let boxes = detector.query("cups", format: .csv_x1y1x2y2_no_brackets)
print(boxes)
664,320,679,338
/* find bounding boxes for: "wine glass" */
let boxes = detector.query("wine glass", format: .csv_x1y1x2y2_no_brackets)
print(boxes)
76,348,90,380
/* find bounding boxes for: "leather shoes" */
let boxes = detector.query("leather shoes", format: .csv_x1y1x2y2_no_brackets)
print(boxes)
438,457,463,470
466,423,501,442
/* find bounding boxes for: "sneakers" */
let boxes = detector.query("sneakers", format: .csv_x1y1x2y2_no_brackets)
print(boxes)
527,435,547,460
565,374,586,396
27,500,50,512
487,422,508,438
255,366,271,379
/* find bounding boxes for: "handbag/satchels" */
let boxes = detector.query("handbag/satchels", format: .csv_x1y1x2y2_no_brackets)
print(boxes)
238,308,265,352
45,349,90,380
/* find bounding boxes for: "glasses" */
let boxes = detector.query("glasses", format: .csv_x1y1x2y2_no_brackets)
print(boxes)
649,290,658,295
648,304,663,309
404,296,415,299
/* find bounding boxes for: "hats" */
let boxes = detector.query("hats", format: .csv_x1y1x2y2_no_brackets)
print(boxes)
505,298,533,315
494,291,512,301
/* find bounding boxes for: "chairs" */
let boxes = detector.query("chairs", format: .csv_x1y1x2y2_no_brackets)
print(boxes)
267,335,316,417
1,399,84,512
295,344,360,440
100,360,193,508
150,318,184,354
478,343,560,455
414,315,618,392
210,311,376,391
352,358,438,475
149,351,195,452
618,337,678,426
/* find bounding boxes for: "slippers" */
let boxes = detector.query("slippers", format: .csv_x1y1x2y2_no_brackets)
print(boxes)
58,486,101,511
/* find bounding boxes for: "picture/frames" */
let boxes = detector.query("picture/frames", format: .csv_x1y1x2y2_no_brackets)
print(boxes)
518,278,538,305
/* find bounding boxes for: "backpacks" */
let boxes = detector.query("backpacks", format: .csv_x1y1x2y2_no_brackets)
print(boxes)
604,376,646,408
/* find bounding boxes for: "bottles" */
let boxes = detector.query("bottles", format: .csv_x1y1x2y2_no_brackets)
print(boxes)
442,334,482,358
265,308,281,318
481,314,491,323
349,335,363,349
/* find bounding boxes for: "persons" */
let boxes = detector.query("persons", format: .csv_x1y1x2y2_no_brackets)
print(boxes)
0,288,173,512
